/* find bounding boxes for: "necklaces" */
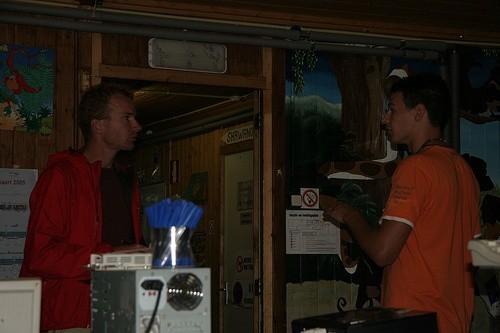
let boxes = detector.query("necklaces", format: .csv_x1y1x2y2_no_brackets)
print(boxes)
416,136,444,153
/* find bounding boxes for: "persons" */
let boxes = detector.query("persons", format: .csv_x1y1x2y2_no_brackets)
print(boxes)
319,74,482,333
18,84,149,332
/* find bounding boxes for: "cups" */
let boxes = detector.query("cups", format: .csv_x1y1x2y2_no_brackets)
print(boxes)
148,227,196,269
